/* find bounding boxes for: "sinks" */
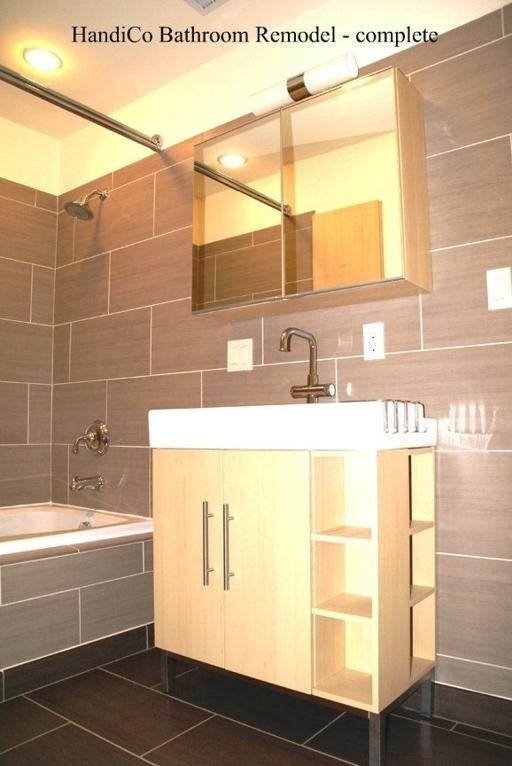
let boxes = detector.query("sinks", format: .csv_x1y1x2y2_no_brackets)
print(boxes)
150,400,439,450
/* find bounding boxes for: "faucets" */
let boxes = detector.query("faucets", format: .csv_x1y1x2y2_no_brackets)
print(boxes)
69,475,104,494
279,325,336,403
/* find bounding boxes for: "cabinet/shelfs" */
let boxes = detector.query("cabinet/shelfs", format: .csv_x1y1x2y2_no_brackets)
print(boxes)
191,65,434,316
150,447,436,761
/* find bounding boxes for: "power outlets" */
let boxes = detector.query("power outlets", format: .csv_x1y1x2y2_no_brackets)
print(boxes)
363,321,385,361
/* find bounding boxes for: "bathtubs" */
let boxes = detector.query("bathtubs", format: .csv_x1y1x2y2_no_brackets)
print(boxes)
0,500,154,708
